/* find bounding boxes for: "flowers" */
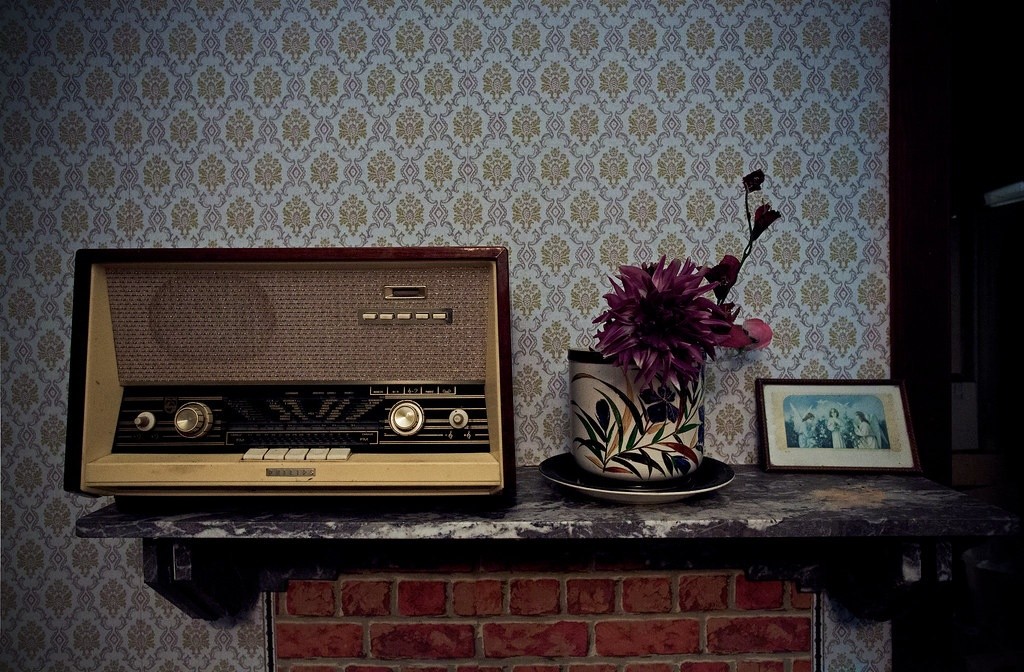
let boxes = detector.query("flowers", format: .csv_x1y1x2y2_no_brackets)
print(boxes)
591,171,782,392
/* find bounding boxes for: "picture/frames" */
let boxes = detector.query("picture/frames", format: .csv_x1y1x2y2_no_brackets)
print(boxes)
756,378,921,473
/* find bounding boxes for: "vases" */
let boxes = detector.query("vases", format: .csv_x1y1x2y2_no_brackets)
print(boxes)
566,348,707,479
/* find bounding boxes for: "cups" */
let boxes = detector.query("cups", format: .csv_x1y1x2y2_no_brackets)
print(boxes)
568,348,705,479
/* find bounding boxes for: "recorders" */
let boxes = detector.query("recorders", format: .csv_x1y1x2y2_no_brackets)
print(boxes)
64,245,518,513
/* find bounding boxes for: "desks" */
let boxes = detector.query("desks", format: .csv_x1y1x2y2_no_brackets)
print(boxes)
76,462,1024,623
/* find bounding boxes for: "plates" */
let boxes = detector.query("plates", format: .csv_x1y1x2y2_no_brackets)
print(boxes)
536,452,735,504
